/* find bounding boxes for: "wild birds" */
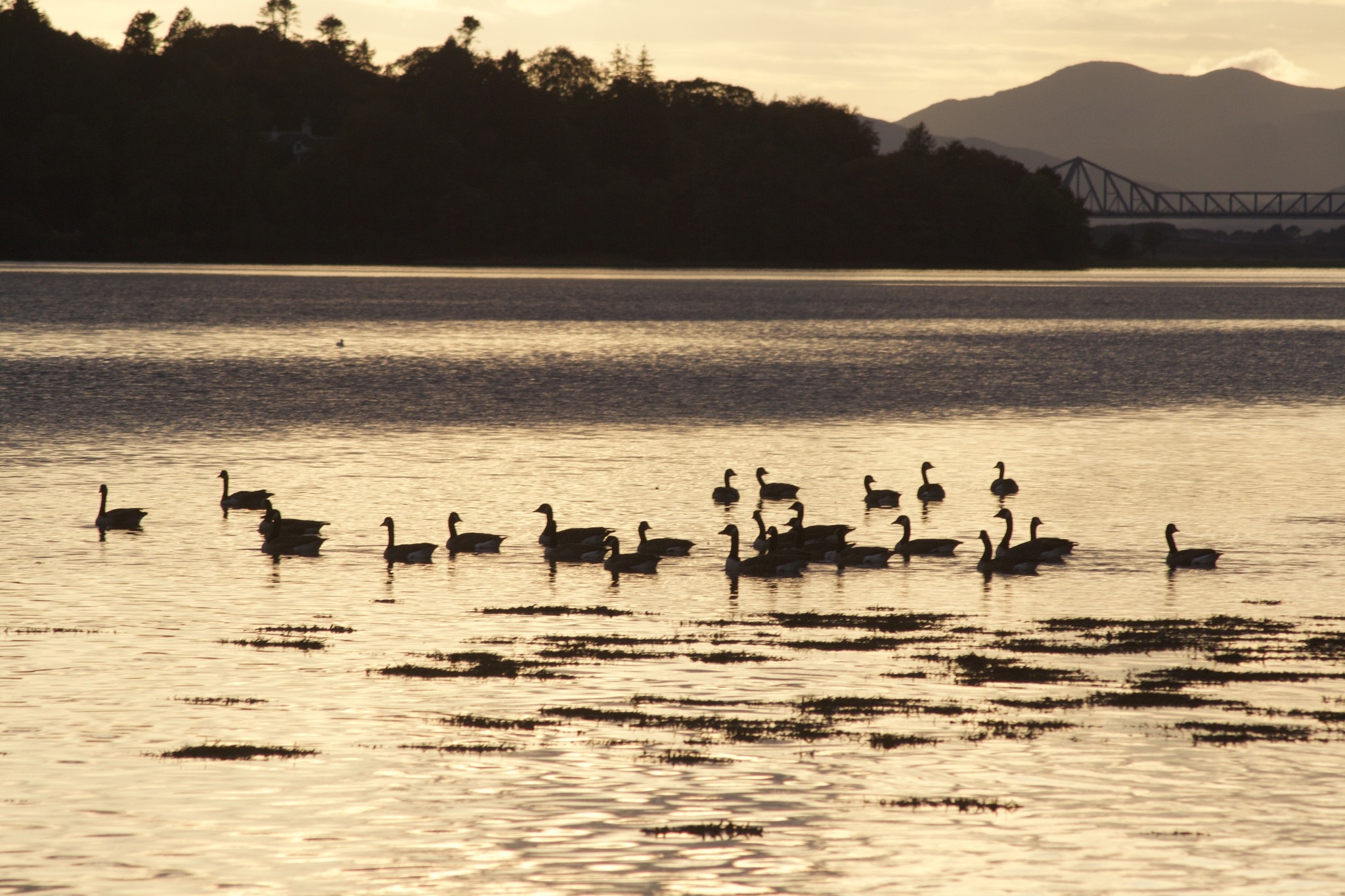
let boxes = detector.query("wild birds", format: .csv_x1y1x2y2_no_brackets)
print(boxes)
532,502,697,573
976,508,1079,571
380,516,439,560
717,502,900,572
891,515,964,555
446,512,511,551
863,474,902,504
990,461,1018,495
96,484,149,529
917,461,945,500
258,498,331,557
217,469,275,509
1166,523,1224,566
712,467,803,502
